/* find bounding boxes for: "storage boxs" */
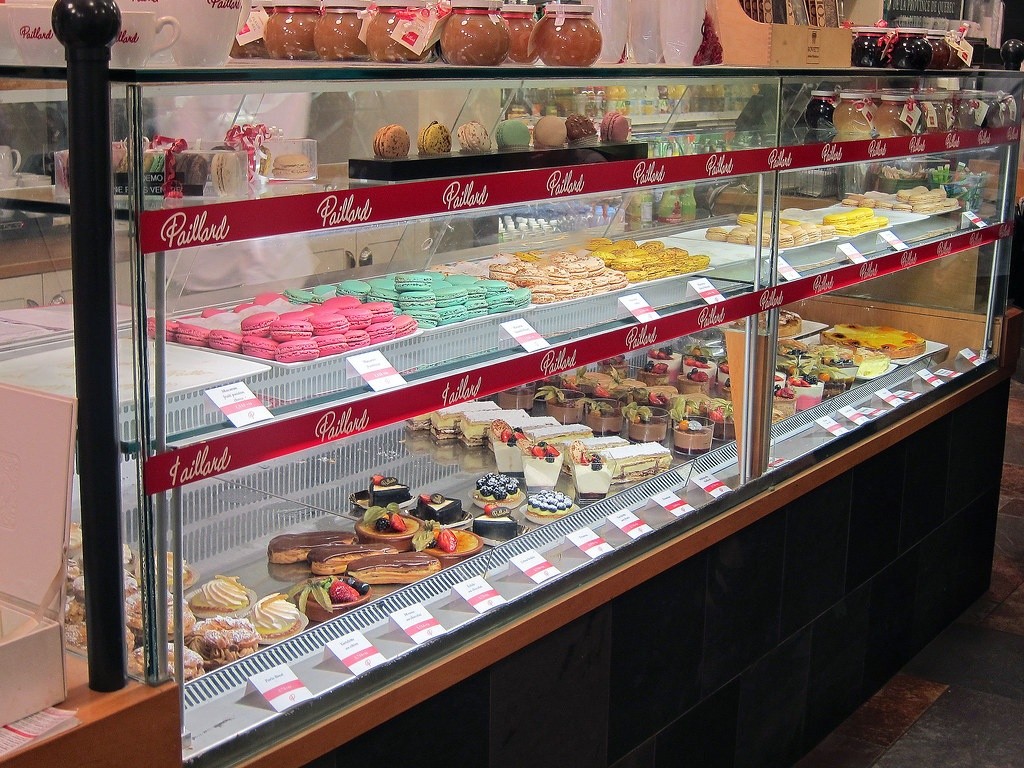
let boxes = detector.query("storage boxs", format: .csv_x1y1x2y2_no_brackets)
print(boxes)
922,170,994,212
708,0,851,67
0,382,78,731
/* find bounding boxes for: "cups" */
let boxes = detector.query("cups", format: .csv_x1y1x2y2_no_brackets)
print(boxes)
581,0,706,68
497,381,715,457
493,432,617,504
0,0,253,71
0,145,52,218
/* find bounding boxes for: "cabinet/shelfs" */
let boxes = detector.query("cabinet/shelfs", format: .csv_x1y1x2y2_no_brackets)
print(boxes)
0,229,148,308
304,218,430,274
0,0,1024,768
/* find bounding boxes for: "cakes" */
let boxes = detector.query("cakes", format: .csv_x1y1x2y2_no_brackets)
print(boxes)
819,325,926,358
717,309,802,337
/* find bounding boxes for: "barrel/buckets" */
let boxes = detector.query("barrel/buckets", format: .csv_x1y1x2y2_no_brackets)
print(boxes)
927,170,989,214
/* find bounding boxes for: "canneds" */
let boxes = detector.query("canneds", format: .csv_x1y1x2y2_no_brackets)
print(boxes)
804,87,1010,136
851,26,962,71
229,0,602,68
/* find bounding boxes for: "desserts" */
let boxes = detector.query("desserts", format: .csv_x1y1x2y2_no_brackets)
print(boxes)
63,473,574,685
113,141,314,193
148,238,712,362
374,113,629,158
706,186,959,248
405,339,890,500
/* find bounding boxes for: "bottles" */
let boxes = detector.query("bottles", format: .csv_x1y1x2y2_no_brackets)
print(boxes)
497,180,697,244
929,161,974,184
500,83,760,121
805,26,1017,136
228,1,604,69
